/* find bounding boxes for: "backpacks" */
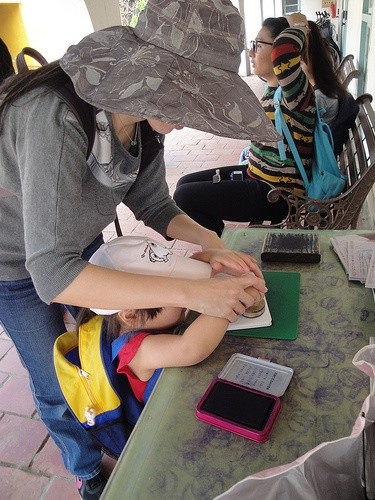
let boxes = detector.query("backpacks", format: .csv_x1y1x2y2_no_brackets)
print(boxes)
273,85,348,213
51,313,162,461
327,90,360,154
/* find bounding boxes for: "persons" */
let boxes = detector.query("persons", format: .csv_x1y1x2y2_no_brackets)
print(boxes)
237,37,339,166
75,235,268,432
2,4,270,500
173,12,348,241
315,18,341,70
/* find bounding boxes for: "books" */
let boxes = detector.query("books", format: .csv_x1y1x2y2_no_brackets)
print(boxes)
224,292,273,332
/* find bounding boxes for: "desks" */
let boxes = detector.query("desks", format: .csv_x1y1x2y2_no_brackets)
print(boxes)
98,224,375,500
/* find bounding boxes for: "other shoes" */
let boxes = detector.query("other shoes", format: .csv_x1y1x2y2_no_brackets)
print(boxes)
75,472,108,500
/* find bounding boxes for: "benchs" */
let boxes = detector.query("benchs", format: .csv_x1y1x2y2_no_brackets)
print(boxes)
334,54,359,88
244,90,375,229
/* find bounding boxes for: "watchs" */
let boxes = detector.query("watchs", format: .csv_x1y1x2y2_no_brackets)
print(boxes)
292,21,311,33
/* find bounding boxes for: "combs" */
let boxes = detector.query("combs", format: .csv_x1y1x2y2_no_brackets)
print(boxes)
212,169,221,183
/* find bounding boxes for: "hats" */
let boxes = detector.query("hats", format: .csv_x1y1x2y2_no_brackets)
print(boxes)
86,235,212,317
59,1,286,144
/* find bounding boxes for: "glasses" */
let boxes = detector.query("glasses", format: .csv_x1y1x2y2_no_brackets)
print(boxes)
250,38,273,53
